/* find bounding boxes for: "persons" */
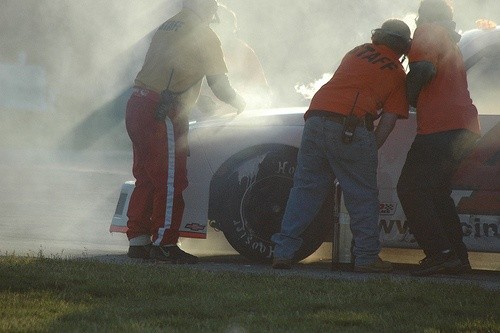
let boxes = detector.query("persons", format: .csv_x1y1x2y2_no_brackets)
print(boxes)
272,19,411,272
407,0,480,276
125,0,247,263
193,5,270,121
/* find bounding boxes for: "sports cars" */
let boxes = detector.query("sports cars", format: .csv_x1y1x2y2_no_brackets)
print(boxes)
109,24,500,264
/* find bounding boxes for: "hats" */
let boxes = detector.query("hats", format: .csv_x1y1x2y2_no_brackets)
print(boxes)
381,19,411,43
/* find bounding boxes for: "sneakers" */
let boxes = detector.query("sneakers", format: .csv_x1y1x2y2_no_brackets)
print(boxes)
150,244,200,264
272,255,292,269
354,256,393,273
127,243,153,259
408,243,472,277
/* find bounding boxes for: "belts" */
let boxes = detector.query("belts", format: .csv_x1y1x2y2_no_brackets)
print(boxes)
305,110,367,128
